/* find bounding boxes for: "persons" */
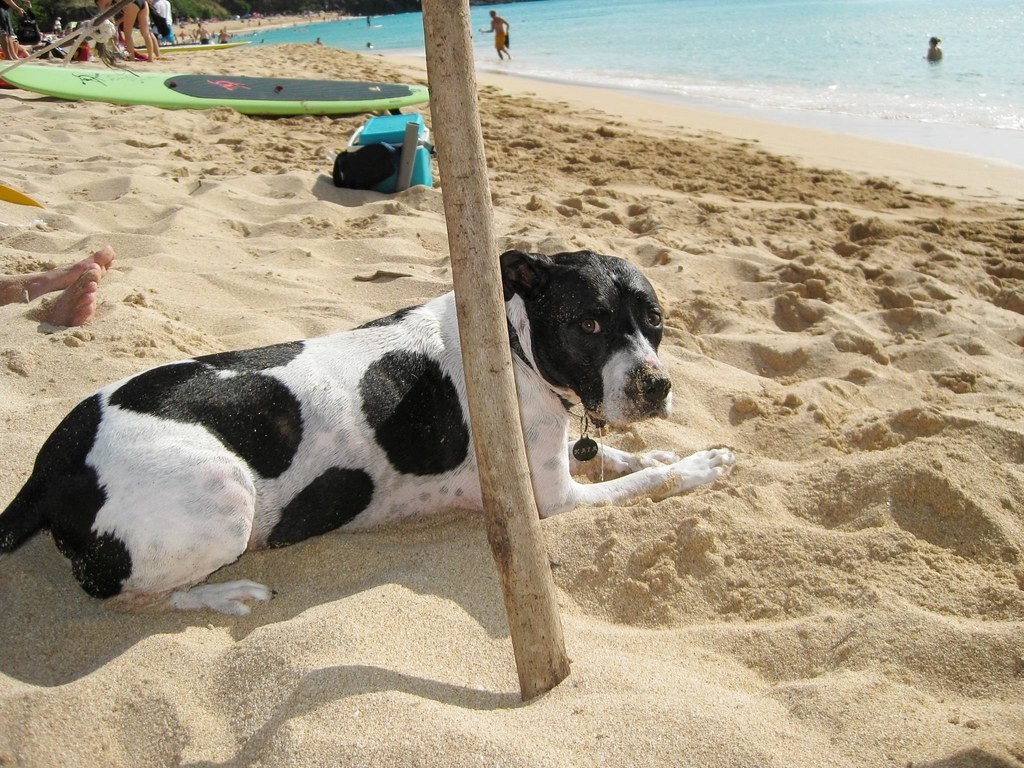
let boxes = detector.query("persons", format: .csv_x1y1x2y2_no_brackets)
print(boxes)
925,36,942,61
172,8,374,50
0,0,31,60
150,0,175,47
113,9,161,58
0,17,93,63
94,0,153,63
479,10,512,60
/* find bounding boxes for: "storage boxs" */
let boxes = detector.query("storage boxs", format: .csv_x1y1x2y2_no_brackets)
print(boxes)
333,113,437,194
73,45,89,61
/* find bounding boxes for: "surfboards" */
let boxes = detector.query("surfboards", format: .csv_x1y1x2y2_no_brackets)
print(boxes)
0,63,432,117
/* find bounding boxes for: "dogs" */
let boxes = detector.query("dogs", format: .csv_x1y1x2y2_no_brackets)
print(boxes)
0,250,738,617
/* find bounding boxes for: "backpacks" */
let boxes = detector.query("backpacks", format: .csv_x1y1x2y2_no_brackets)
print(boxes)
14,10,41,44
